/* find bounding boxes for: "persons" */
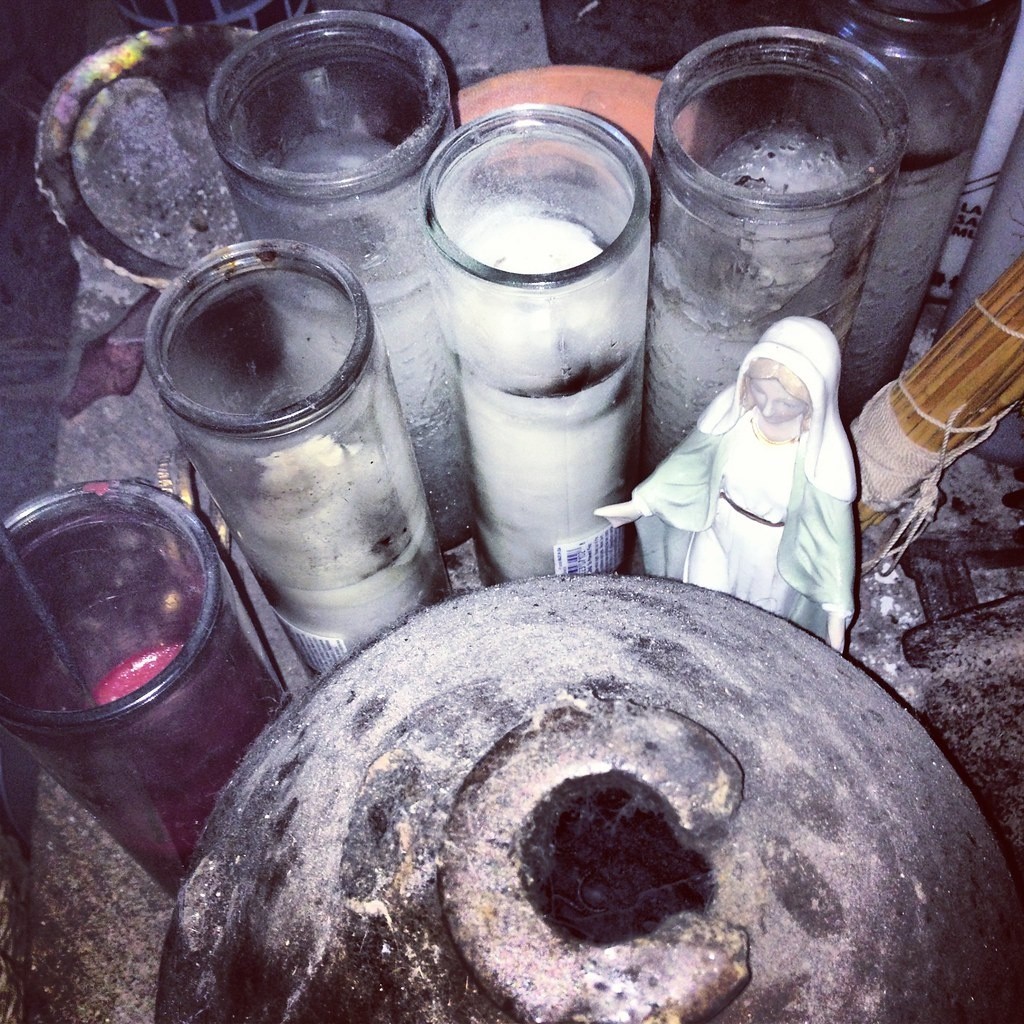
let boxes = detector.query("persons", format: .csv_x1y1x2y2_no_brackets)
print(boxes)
592,314,859,653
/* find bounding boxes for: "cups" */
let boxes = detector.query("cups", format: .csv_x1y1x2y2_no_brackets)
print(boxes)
143,237,451,681
827,0,1024,423
420,101,658,581
0,478,287,904
652,24,909,479
203,10,473,557
